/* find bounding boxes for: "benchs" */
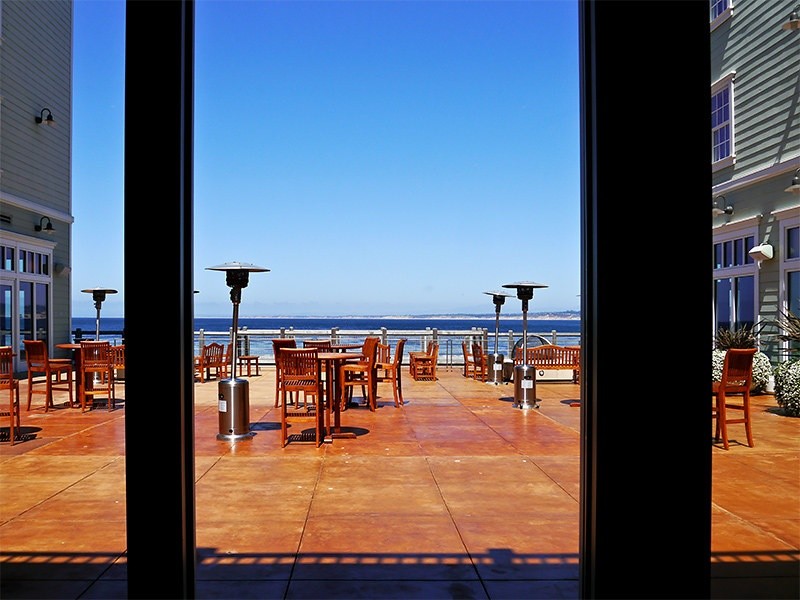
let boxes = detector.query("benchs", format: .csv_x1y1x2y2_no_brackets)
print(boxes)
516,345,581,384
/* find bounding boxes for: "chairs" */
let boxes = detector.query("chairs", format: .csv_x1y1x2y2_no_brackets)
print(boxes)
209,342,238,378
23,340,73,412
193,341,224,383
80,340,115,414
279,347,324,448
711,347,758,450
407,340,439,381
0,346,20,445
461,342,490,383
270,337,408,413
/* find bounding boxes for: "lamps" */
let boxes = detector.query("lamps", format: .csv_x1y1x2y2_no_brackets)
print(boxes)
54,262,71,274
34,216,56,235
35,107,56,126
782,4,800,32
748,243,774,261
783,167,800,197
712,204,735,219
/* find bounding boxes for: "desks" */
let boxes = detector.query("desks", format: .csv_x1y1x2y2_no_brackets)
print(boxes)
304,344,363,407
54,343,112,408
291,352,364,440
239,355,259,377
